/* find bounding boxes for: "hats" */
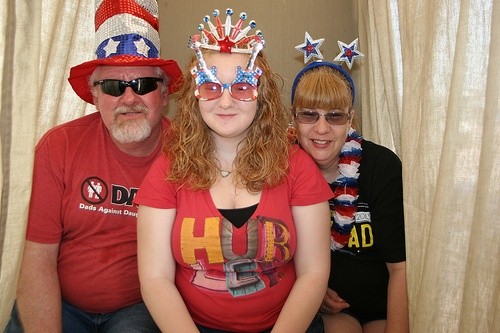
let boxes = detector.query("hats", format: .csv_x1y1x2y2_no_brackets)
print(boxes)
68,0,184,105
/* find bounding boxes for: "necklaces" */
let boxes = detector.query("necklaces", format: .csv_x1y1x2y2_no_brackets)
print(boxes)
288,124,363,250
211,157,243,178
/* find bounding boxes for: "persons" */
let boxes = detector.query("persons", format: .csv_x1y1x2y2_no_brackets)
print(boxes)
289,65,410,333
131,35,336,332
3,46,173,333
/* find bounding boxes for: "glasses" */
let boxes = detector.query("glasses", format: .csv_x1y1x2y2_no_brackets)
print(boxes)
93,77,163,97
197,81,257,102
295,110,351,125
